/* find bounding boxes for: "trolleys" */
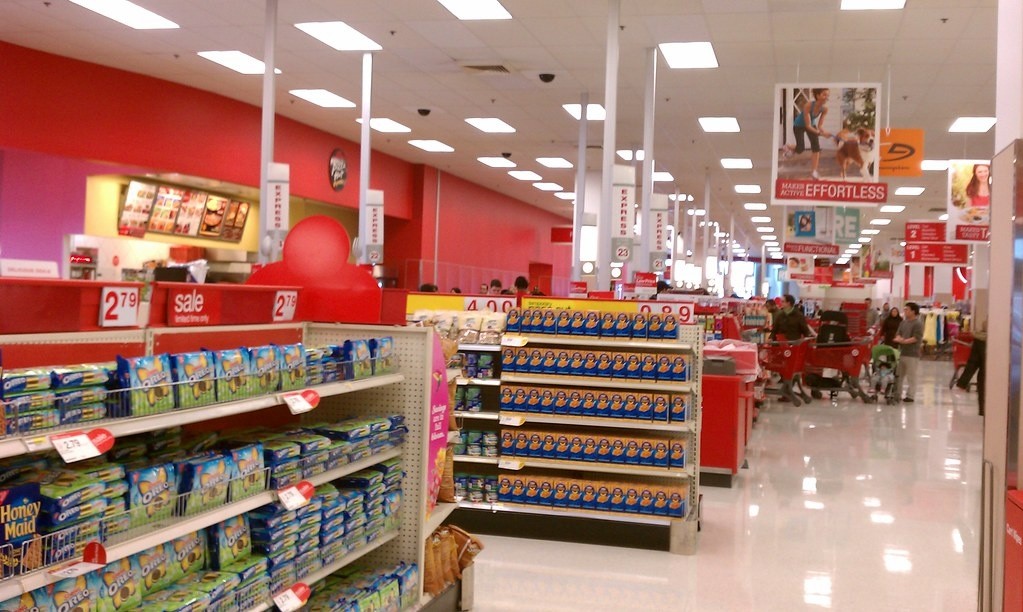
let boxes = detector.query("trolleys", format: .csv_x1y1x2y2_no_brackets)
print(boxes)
805,336,874,404
756,337,811,407
948,332,979,393
848,326,881,387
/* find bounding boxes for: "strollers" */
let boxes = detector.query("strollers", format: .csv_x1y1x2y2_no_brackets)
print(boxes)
869,341,901,405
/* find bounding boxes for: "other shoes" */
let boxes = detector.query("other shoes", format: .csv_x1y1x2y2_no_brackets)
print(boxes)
811,170,819,180
868,388,875,392
903,397,913,402
880,388,885,394
778,396,791,402
783,143,794,160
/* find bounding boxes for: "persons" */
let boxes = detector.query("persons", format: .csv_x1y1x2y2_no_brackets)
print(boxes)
765,294,823,401
648,281,673,299
895,302,925,404
420,284,438,292
867,362,895,395
449,287,462,294
864,297,891,330
477,275,546,295
783,88,829,180
966,164,992,208
880,307,903,349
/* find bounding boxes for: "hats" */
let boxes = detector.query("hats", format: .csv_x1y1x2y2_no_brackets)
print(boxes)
657,281,672,289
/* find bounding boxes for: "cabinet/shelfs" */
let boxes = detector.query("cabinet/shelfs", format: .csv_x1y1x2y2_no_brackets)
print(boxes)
498,335,699,553
452,345,504,509
0,322,472,612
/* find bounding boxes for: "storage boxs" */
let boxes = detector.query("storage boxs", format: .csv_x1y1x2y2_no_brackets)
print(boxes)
499,306,693,519
